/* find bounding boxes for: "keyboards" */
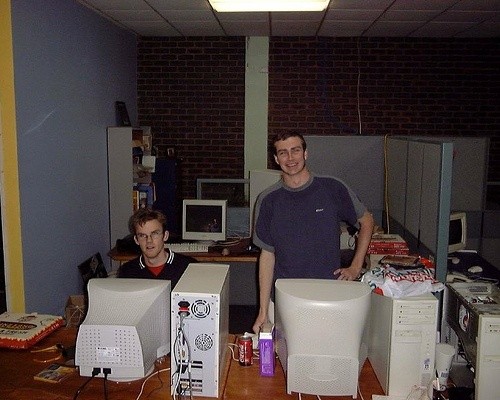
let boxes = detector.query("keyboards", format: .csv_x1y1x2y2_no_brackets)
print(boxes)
164,243,209,253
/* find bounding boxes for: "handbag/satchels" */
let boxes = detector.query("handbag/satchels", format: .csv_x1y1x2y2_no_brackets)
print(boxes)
362,264,443,299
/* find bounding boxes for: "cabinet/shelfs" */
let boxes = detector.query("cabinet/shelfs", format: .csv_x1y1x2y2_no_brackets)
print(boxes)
107,126,152,270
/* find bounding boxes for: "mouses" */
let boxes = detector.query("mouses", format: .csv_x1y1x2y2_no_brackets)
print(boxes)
468,266,482,273
222,248,230,255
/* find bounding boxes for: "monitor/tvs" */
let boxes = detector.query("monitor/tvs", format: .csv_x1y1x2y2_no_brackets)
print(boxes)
447,212,468,255
274,278,372,398
182,200,225,246
75,278,173,383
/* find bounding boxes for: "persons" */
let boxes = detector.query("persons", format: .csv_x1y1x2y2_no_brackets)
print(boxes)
118,208,198,291
252,132,375,335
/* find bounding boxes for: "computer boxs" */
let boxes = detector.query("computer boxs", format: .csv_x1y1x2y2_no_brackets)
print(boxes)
170,263,230,398
368,291,440,400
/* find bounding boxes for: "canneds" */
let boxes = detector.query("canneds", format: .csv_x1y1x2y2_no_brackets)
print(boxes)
238,336,253,366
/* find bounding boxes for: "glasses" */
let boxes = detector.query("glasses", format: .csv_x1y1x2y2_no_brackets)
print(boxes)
136,231,164,242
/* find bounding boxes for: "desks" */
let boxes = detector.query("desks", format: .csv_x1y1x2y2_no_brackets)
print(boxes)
106,238,259,266
0,327,386,400
